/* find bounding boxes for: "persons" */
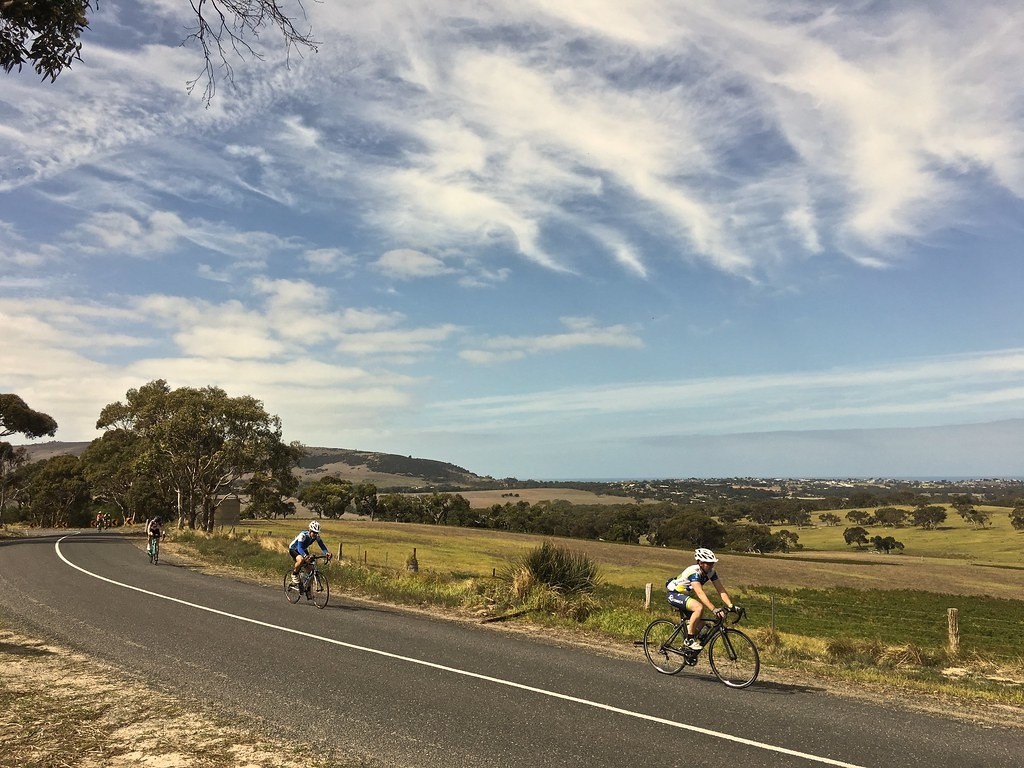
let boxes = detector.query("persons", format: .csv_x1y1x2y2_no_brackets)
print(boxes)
289,521,332,584
147,516,166,551
666,548,740,649
97,511,111,531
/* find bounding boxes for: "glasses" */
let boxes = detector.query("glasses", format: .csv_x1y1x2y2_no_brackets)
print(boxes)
703,561,714,565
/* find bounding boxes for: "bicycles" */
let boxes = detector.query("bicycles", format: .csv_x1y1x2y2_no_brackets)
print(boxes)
146,533,162,565
643,605,760,689
284,554,333,609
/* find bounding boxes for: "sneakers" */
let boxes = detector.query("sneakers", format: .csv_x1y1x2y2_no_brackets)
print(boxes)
291,573,300,584
308,594,314,598
683,637,703,650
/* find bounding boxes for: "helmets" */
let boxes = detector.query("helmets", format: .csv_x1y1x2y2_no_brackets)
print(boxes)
694,548,719,562
309,520,321,532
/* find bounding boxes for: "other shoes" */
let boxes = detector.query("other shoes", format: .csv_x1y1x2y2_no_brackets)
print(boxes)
146,544,151,550
